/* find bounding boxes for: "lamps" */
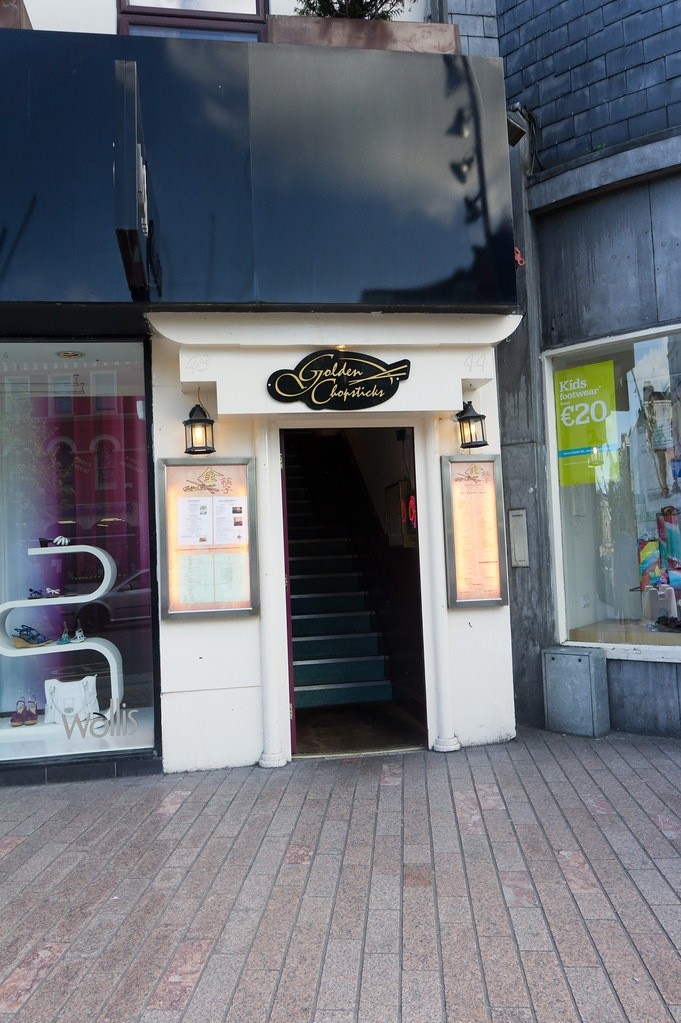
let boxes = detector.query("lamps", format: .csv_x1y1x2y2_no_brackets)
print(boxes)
182,404,215,455
443,54,490,228
456,401,489,448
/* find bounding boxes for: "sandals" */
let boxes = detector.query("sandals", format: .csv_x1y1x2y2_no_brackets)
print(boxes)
70,629,87,643
56,629,71,645
671,487,681,492
660,490,669,496
661,506,681,516
45,587,59,597
27,589,45,599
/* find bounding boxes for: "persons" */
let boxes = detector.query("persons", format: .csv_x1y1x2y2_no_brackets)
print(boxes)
649,381,681,497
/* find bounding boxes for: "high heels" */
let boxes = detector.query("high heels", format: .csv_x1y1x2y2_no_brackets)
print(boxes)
39,536,74,547
12,628,47,649
20,625,52,643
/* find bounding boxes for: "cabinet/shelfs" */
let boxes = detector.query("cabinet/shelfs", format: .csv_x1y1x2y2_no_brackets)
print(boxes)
0,544,123,738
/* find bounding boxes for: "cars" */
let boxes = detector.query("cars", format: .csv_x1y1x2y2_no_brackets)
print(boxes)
62,567,152,631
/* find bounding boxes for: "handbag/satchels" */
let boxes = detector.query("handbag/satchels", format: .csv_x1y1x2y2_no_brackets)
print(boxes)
668,455,681,478
44,674,101,725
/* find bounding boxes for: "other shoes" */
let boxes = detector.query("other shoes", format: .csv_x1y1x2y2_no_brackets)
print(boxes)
656,615,681,633
10,697,27,726
62,586,78,596
24,695,38,725
